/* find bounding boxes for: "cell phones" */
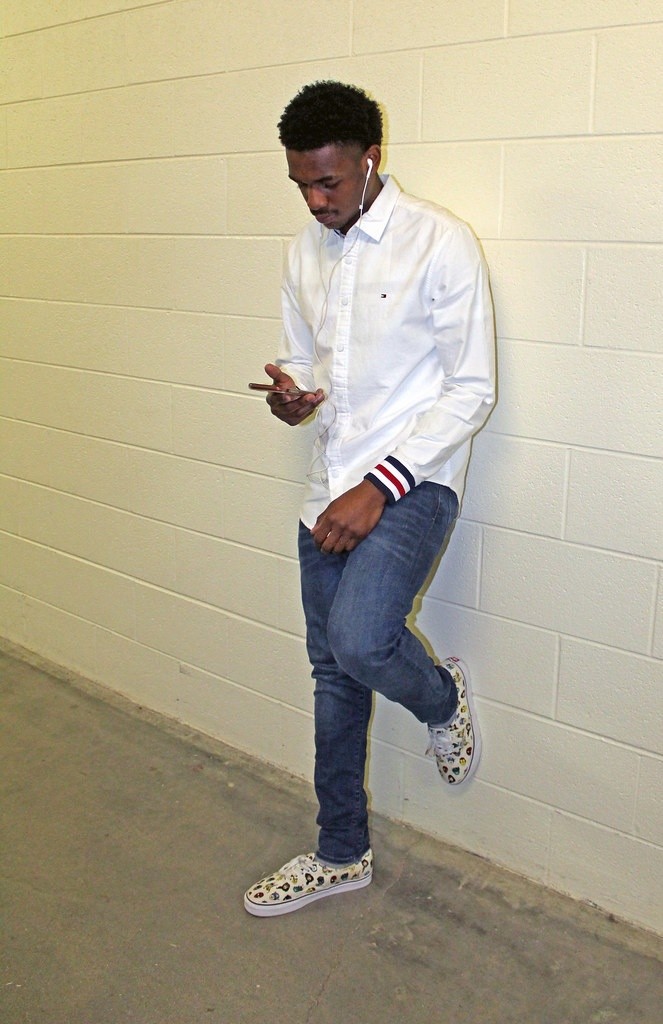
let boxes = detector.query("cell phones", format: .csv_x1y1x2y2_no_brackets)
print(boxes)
248,384,317,397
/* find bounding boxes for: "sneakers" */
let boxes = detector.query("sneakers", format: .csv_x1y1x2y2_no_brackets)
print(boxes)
425,655,481,787
243,849,373,917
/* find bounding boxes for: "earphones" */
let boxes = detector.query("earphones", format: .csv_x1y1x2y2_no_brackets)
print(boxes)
366,158,373,179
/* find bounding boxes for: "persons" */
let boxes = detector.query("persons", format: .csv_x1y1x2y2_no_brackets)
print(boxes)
241,79,503,920
